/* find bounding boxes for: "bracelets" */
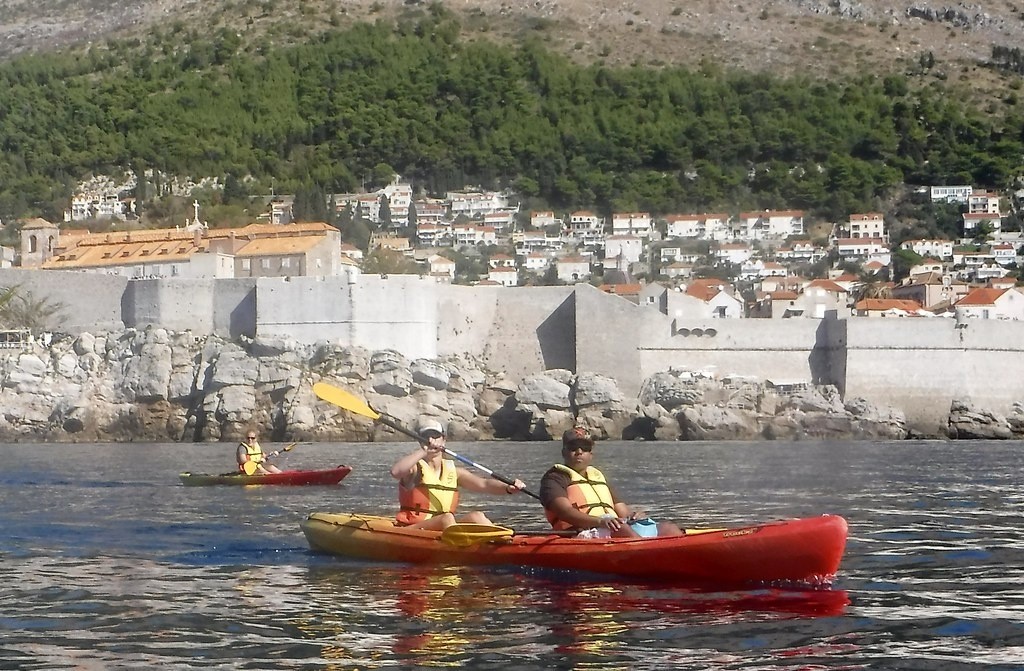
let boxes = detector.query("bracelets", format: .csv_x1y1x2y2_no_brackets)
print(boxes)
506,485,513,494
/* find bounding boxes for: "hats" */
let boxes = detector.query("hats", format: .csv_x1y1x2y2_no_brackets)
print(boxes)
420,419,442,434
563,427,593,446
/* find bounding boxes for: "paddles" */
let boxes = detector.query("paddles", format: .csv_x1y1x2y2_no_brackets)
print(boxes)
244,441,298,476
311,383,541,500
441,523,730,548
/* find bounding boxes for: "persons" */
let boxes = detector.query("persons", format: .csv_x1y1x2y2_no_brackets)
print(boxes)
391,420,526,531
540,426,683,540
237,430,283,474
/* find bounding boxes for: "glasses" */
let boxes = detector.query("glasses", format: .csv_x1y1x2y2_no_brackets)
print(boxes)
422,432,441,439
564,443,592,452
247,437,255,439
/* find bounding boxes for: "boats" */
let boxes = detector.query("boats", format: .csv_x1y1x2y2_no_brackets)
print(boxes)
299,510,848,586
176,464,352,486
